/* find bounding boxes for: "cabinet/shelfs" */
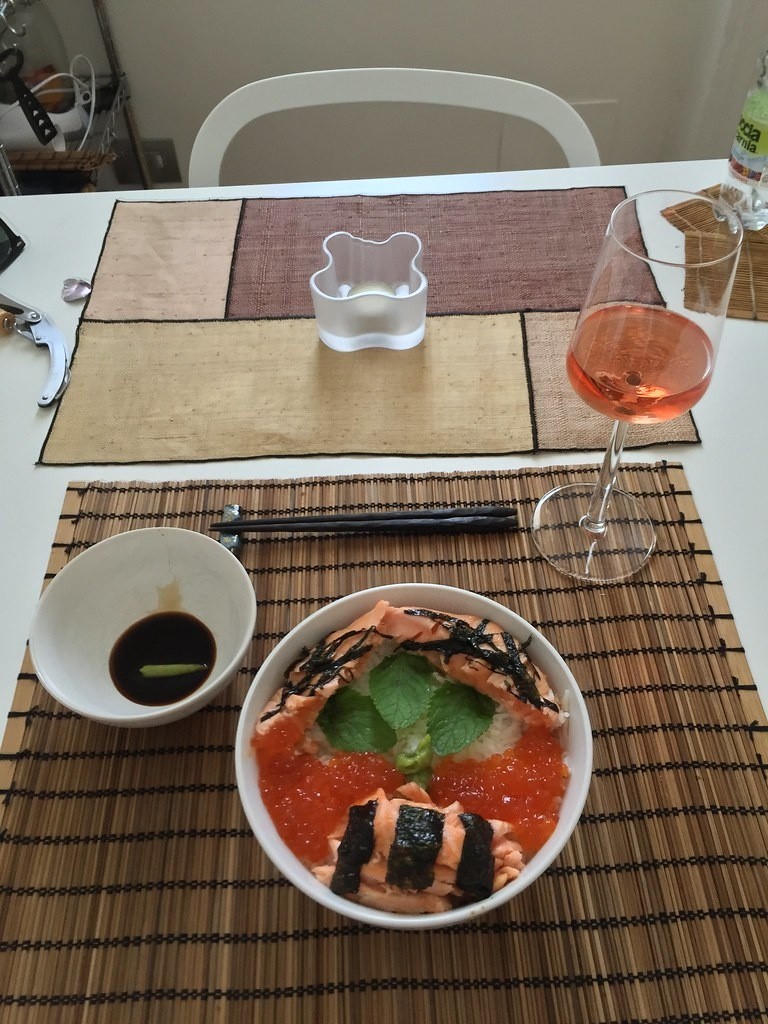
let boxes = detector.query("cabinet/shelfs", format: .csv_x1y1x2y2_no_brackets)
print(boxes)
0,0,155,197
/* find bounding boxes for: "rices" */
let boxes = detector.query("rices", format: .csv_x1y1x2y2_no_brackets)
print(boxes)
286,634,525,763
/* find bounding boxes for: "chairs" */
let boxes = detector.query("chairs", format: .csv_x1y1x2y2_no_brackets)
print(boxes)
188,67,602,188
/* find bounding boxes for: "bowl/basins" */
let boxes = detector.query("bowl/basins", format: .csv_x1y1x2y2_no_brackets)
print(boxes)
28,527,259,726
233,583,596,933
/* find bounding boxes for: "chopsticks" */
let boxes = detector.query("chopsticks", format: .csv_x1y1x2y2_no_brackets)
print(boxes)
206,508,521,532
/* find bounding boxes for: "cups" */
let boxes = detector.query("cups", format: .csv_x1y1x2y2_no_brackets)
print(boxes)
309,231,429,353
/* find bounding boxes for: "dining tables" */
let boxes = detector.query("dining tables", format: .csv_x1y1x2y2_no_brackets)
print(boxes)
0,157,767,1023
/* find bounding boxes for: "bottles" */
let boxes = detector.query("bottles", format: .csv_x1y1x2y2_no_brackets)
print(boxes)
715,48,768,235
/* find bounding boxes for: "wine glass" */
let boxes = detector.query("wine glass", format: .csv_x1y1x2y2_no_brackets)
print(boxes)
528,189,742,581
